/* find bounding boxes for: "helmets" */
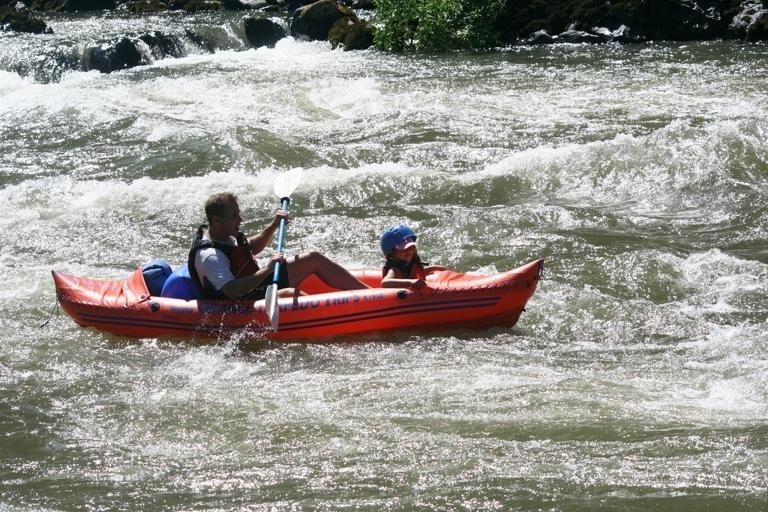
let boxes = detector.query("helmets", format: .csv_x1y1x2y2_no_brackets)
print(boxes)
380,226,417,257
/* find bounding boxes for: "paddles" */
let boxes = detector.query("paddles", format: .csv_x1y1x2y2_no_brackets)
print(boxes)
264,167,301,332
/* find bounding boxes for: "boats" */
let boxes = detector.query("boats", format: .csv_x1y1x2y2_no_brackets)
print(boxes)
47,254,543,353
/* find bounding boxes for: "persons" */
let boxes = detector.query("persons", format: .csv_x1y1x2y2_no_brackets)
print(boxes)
379,225,427,289
188,192,373,301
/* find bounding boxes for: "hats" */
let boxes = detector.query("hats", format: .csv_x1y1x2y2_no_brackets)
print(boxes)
395,237,417,250
